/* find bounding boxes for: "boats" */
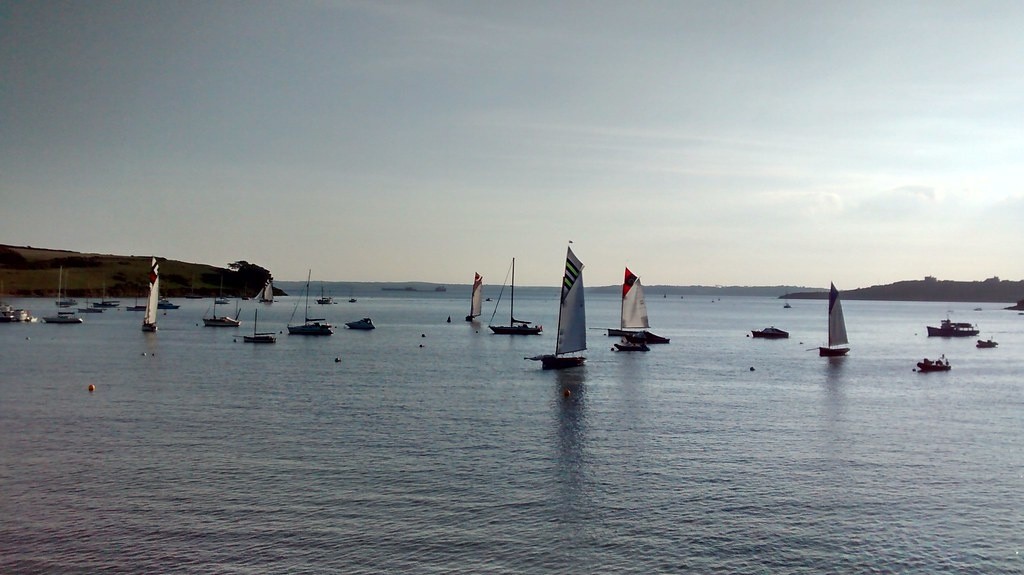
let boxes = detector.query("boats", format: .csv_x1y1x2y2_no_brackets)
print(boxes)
155,291,180,309
345,318,376,330
381,286,417,291
127,293,147,311
916,355,952,372
0,303,38,322
751,327,789,339
975,338,998,349
614,342,651,351
1004,298,1024,310
927,318,980,336
348,299,357,302
622,331,670,344
185,291,202,298
784,302,791,308
435,285,446,291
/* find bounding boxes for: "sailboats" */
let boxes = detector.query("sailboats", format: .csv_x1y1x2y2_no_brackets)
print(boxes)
55,273,78,305
488,258,543,335
202,294,242,326
93,283,119,308
606,267,650,337
41,266,83,324
242,307,277,343
287,269,334,335
316,281,334,304
141,257,160,331
820,281,850,357
255,278,275,303
521,242,589,370
213,275,230,303
464,272,485,322
78,290,103,314
